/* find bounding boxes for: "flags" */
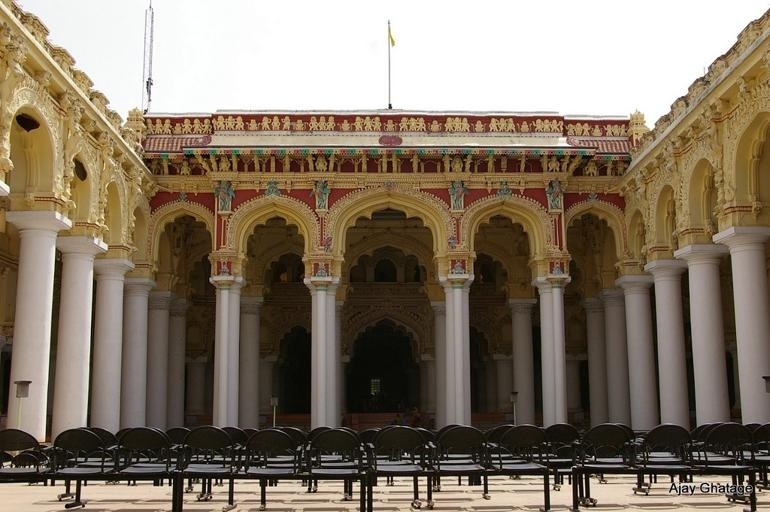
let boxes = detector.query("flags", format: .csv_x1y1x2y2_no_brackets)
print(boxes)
387,26,395,46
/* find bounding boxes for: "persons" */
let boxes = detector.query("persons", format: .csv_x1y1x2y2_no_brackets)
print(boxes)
341,409,350,428
409,407,421,428
